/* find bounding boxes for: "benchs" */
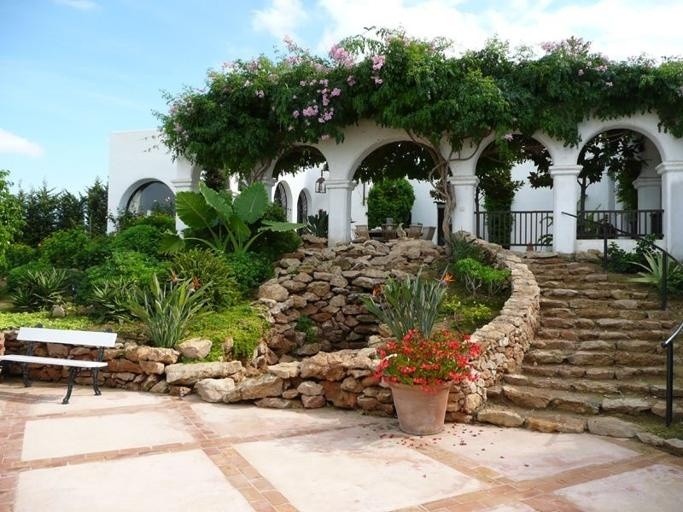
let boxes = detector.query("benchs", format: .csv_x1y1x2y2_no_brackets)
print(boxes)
0,327,118,403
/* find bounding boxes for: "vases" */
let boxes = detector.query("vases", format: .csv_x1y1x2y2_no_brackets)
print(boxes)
388,382,451,436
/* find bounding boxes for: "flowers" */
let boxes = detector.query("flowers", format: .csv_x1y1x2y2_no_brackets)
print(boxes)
373,329,480,394
